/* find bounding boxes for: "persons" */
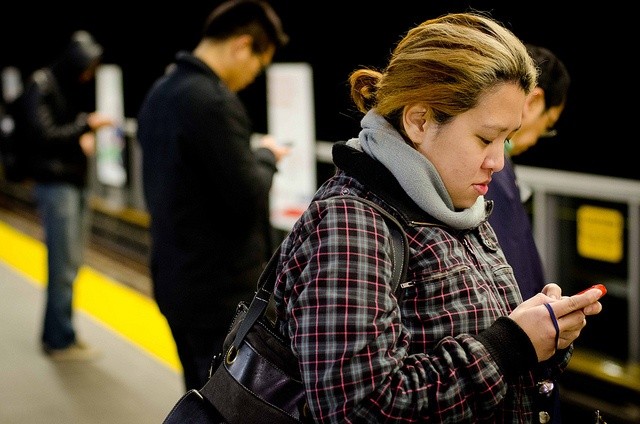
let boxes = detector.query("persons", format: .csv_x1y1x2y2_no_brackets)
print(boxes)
484,46,568,424
167,13,606,424
138,0,290,391
2,31,116,363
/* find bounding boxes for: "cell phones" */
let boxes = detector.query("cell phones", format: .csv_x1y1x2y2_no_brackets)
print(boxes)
575,284,607,297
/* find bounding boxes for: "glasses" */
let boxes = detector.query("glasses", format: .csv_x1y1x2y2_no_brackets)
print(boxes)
538,111,557,137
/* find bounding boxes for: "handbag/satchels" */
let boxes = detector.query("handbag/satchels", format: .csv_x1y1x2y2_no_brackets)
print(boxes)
162,194,411,423
